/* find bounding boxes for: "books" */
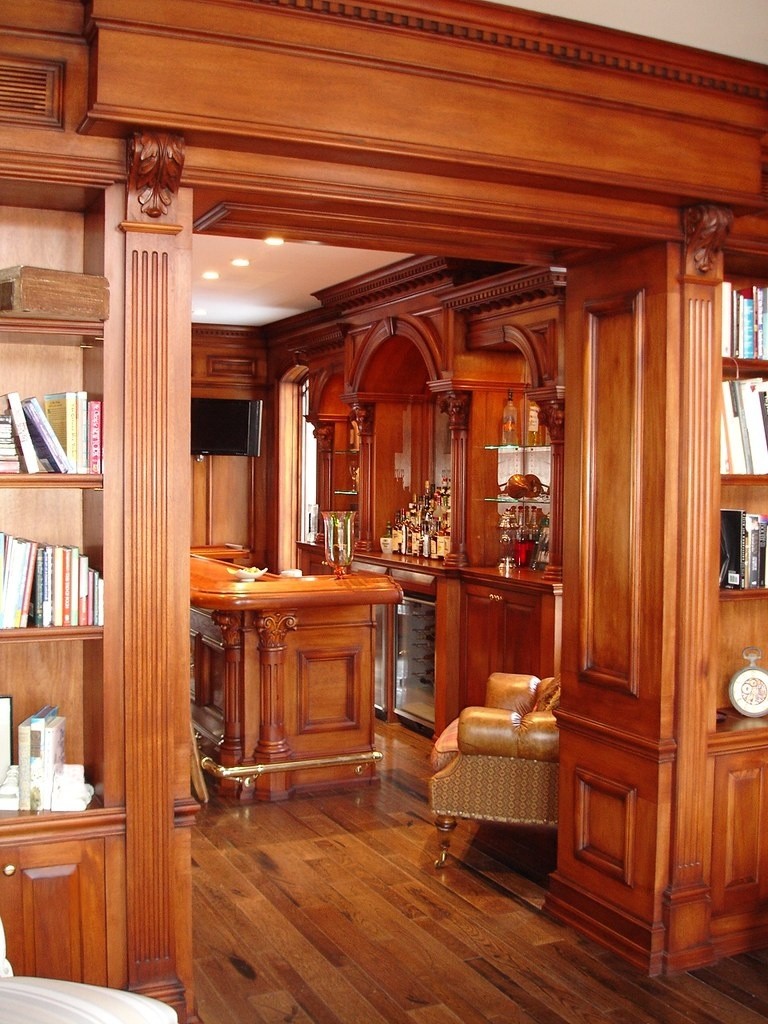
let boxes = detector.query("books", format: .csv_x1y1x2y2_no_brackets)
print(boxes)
0,264,111,813
720,282,768,590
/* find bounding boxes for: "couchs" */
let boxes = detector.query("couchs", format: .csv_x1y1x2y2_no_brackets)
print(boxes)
430,672,561,868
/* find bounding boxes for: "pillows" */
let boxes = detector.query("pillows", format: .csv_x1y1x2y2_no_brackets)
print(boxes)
534,673,561,713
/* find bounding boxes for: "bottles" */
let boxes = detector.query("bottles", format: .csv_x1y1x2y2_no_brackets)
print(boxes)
511,505,549,570
380,477,451,560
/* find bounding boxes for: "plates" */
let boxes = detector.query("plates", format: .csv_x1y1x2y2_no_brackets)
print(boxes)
227,568,269,581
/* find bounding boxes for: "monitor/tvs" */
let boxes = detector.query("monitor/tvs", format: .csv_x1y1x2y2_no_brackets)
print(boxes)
190,398,262,457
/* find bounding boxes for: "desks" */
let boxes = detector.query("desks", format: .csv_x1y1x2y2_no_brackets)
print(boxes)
190,543,403,801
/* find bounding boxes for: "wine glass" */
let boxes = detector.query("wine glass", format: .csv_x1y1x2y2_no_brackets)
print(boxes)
321,511,356,580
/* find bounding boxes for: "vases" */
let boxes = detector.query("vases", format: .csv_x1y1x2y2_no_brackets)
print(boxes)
320,511,359,580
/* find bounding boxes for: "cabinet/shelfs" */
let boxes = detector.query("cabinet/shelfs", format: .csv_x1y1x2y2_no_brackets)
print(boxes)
296,265,564,740
0,166,128,1001
704,241,768,954
